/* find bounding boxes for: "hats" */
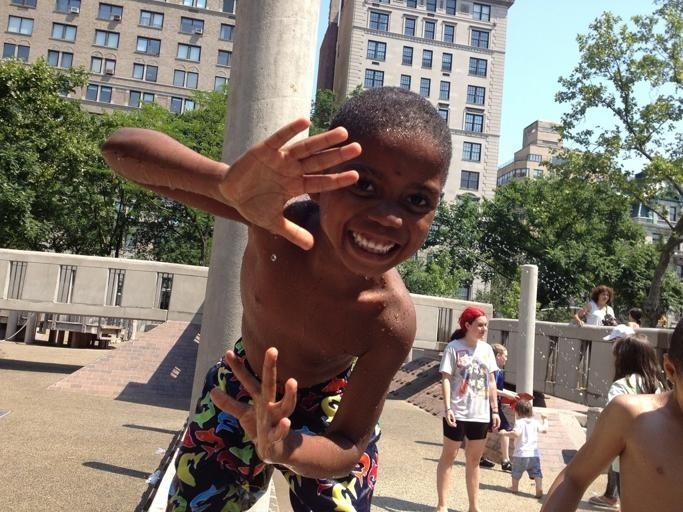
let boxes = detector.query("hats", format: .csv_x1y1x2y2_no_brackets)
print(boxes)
459,307,486,330
602,323,635,341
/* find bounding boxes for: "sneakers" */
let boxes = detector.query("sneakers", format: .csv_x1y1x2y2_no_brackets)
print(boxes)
589,495,619,508
479,456,496,467
502,460,512,471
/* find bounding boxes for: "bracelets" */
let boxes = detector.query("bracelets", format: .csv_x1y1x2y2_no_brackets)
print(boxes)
491,411,498,414
443,407,451,411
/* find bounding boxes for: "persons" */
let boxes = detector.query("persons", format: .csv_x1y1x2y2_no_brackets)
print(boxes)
624,306,643,329
97,85,451,512
586,331,669,508
497,398,548,498
435,305,503,512
475,342,520,473
573,284,614,328
538,313,682,511
